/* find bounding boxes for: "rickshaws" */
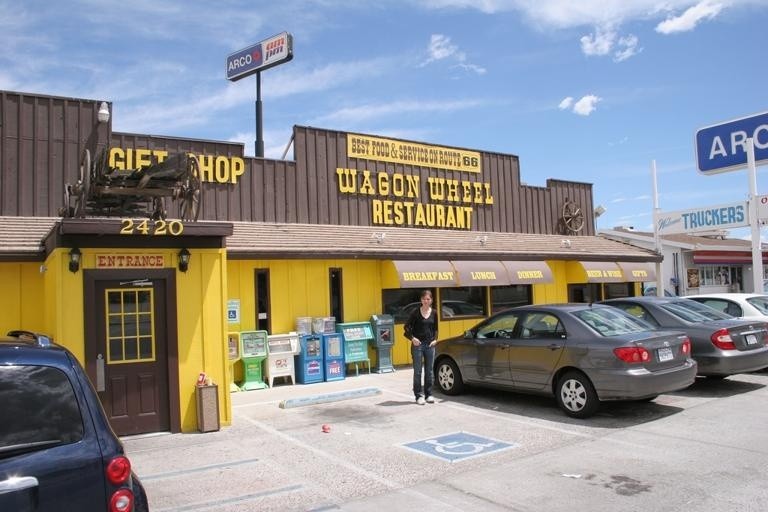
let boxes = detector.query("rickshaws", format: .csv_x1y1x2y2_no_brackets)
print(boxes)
67,148,205,221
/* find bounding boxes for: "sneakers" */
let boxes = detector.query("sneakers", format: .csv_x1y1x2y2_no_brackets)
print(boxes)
426,395,434,403
416,396,426,405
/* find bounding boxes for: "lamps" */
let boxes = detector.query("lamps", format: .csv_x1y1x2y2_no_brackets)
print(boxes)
178,246,191,275
68,246,82,275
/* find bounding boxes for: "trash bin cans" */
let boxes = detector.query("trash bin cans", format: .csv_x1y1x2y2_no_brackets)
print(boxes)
195,384,219,433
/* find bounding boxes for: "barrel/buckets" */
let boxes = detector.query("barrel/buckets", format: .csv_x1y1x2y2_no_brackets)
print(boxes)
295,317,313,336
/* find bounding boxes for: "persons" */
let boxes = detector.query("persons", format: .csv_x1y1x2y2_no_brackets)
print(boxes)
402,291,439,407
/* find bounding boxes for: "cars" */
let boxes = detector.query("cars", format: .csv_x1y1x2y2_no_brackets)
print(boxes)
688,294,768,317
642,285,674,298
596,295,768,384
399,300,482,317
427,300,699,418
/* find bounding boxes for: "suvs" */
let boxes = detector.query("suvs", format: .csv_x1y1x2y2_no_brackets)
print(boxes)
0,330,151,512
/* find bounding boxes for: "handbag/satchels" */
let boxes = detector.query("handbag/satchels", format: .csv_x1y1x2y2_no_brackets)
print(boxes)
404,308,419,341
